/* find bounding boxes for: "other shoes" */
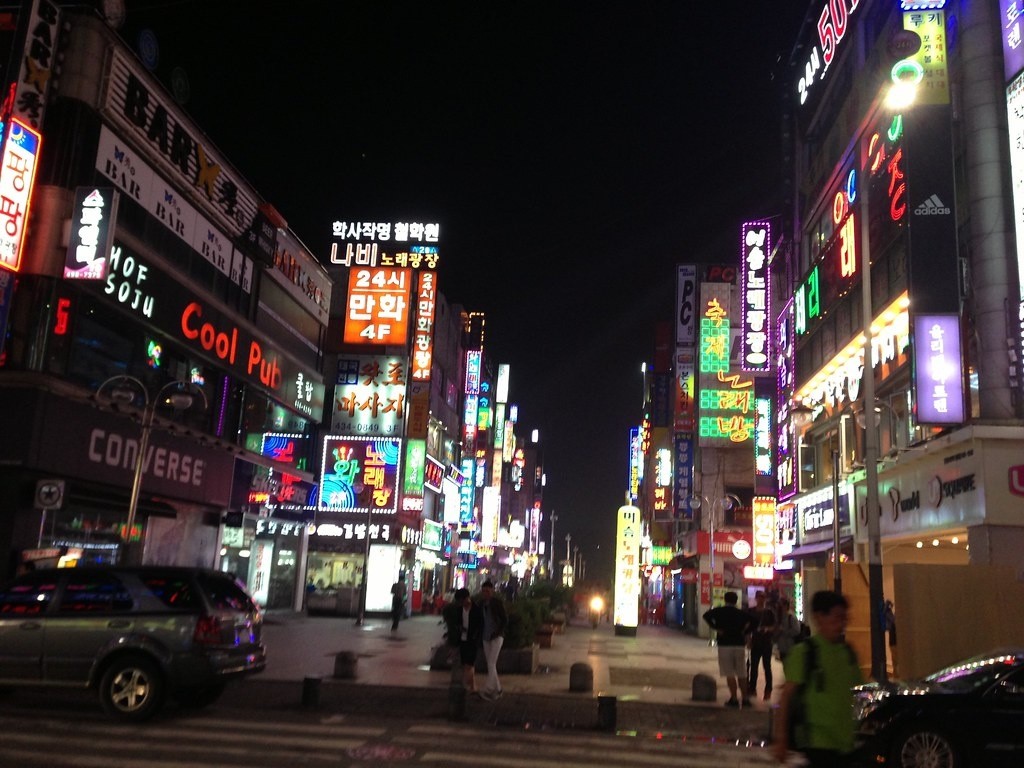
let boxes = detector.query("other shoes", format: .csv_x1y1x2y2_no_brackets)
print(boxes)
764,692,772,701
724,697,753,709
467,689,476,697
494,690,504,700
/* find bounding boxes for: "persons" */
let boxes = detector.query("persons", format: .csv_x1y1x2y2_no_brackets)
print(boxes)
390,576,406,631
889,614,900,679
500,582,521,603
448,578,507,702
420,583,439,616
441,588,456,623
774,590,864,768
703,591,799,708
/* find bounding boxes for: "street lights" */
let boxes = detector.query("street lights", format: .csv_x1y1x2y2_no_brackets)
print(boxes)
836,399,901,683
682,492,740,645
89,375,209,562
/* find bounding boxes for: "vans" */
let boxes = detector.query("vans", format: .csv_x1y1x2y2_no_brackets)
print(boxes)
0,566,268,721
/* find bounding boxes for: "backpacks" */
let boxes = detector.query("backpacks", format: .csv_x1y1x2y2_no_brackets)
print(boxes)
769,640,855,751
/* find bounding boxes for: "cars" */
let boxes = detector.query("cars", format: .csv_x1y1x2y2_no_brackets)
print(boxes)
850,649,1024,768
